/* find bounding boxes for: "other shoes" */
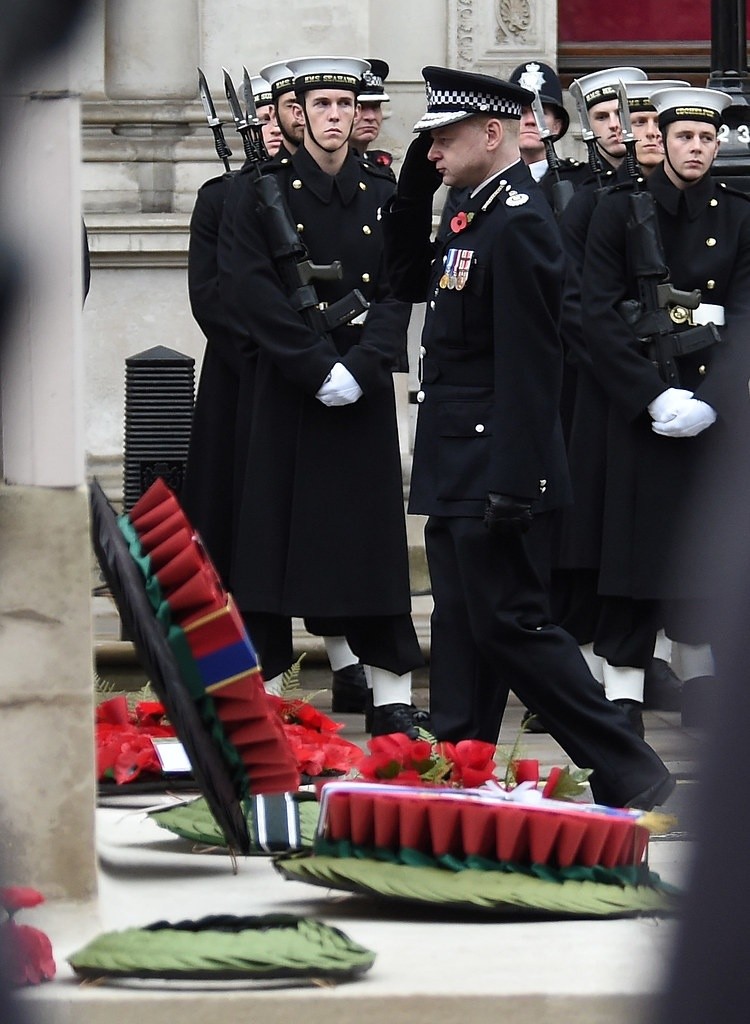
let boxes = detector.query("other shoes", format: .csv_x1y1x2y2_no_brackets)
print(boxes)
611,698,646,740
365,699,432,733
332,663,373,713
521,709,549,731
372,704,422,740
624,772,677,813
682,675,717,732
643,657,684,713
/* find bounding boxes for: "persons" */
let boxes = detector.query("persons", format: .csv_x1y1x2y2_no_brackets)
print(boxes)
184,56,749,813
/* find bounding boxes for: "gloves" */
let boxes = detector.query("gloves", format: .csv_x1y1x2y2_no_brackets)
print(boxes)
315,362,361,406
649,388,716,438
397,131,443,201
483,492,534,545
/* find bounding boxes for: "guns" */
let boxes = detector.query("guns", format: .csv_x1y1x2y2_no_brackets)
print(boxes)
618,77,724,392
576,78,606,199
195,63,231,169
242,67,372,343
531,88,576,212
220,65,253,164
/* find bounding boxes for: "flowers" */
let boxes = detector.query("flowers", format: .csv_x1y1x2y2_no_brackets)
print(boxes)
95,476,692,919
0,886,57,988
450,211,475,234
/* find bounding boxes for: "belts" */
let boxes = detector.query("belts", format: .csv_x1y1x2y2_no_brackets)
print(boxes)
315,301,370,326
686,302,726,327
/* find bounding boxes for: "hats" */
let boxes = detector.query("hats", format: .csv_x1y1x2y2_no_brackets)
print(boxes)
259,59,296,102
285,56,371,96
411,66,535,133
608,80,693,113
356,58,390,102
650,86,734,130
570,67,648,113
510,61,570,142
238,76,274,109
